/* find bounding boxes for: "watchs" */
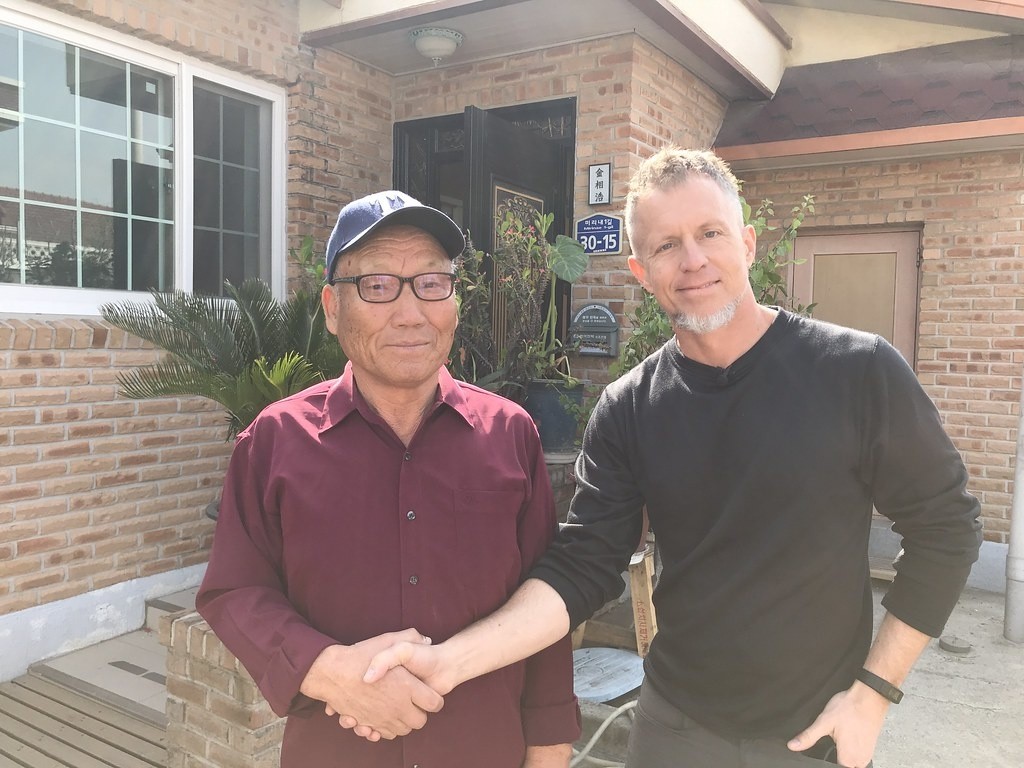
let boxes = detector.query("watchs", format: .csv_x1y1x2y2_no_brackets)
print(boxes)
856,668,904,704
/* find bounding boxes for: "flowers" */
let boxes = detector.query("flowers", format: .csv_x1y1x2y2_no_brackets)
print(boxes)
500,209,588,387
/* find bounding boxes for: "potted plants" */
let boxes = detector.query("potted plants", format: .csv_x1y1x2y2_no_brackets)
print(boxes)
101,278,333,520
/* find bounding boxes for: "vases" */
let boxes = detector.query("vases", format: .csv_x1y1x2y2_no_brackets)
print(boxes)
508,376,591,456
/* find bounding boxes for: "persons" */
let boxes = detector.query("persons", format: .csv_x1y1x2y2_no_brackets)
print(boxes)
326,149,985,768
196,190,583,768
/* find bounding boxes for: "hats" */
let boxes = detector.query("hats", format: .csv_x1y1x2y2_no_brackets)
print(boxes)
325,189,466,286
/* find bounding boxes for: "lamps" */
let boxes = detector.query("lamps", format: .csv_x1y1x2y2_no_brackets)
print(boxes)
409,26,464,67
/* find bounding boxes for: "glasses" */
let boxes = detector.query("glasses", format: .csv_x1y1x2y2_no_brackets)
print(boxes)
333,272,457,303
